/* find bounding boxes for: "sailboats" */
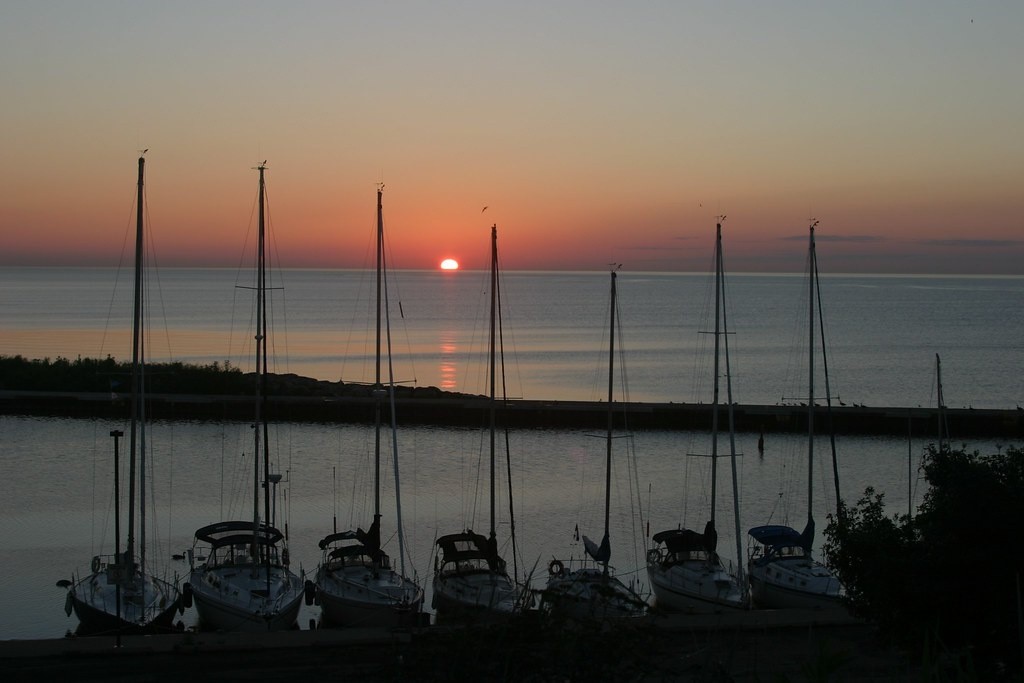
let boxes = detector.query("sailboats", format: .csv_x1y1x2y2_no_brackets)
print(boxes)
644,227,753,610
743,226,844,606
431,221,553,620
313,185,426,625
907,353,954,518
187,164,311,633
542,270,656,616
56,156,181,633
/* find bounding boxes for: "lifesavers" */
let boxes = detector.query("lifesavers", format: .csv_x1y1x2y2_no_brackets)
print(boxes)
206,571,216,587
646,549,661,564
218,553,232,567
90,555,101,572
779,546,792,555
548,559,565,577
